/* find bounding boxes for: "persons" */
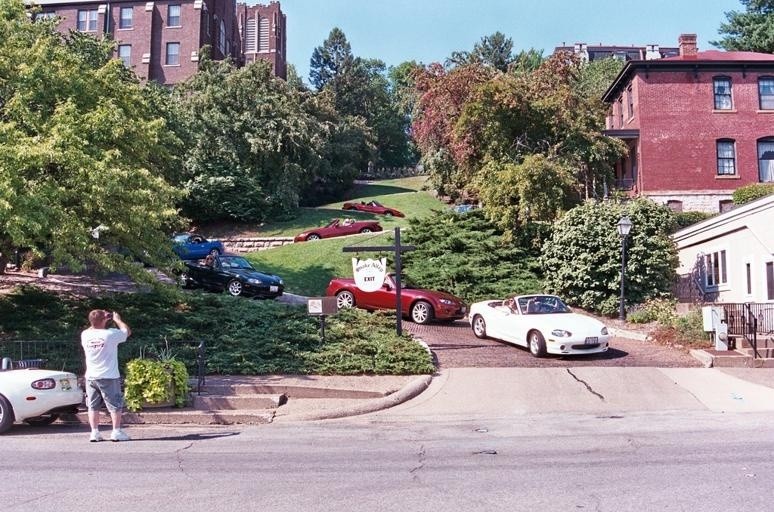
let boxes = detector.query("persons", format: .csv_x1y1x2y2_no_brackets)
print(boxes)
508,297,518,314
206,255,214,267
81,309,131,442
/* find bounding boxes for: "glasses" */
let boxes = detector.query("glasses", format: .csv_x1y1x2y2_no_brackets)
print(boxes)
509,299,513,302
207,258,213,260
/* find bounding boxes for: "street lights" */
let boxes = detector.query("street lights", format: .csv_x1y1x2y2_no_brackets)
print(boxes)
614,217,633,320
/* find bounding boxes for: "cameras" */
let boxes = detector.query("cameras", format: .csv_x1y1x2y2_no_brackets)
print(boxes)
108,313,113,320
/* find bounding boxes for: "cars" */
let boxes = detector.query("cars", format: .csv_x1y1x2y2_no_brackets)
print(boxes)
326,272,609,358
294,218,383,243
0,357,82,434
342,200,405,218
142,233,285,299
456,205,480,213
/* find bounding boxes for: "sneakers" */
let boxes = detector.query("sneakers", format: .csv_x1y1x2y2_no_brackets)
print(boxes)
89,429,130,442
